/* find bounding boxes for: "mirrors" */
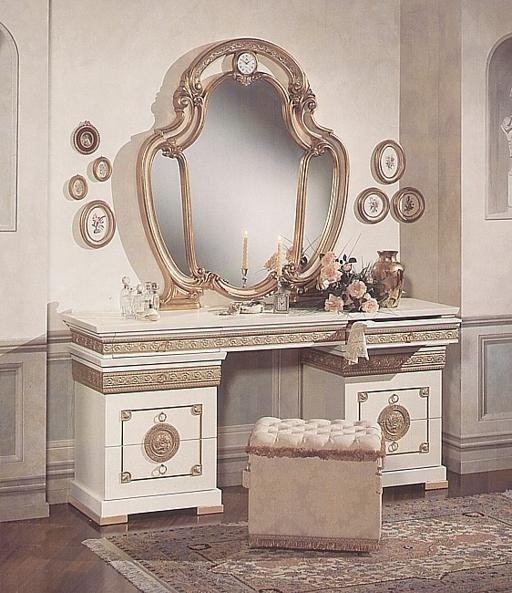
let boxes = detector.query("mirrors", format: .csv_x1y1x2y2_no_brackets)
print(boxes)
139,38,349,311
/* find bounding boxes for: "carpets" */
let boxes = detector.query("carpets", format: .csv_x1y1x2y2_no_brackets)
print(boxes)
82,490,511,593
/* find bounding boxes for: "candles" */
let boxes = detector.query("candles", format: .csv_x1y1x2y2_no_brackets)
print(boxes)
278,241,282,276
243,237,248,269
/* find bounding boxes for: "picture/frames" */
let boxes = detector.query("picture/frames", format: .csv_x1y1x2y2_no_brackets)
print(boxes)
69,122,116,248
356,140,424,223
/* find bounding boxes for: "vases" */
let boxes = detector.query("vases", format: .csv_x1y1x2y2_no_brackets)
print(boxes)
372,251,406,307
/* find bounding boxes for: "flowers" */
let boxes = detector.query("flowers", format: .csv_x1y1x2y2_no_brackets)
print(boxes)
313,251,388,313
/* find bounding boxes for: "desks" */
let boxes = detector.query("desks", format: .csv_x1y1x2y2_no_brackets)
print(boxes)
61,298,461,525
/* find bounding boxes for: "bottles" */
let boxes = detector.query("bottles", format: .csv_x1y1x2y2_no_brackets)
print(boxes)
274,292,290,313
119,282,160,320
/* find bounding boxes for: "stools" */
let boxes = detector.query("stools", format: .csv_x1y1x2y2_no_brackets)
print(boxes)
247,416,382,552
